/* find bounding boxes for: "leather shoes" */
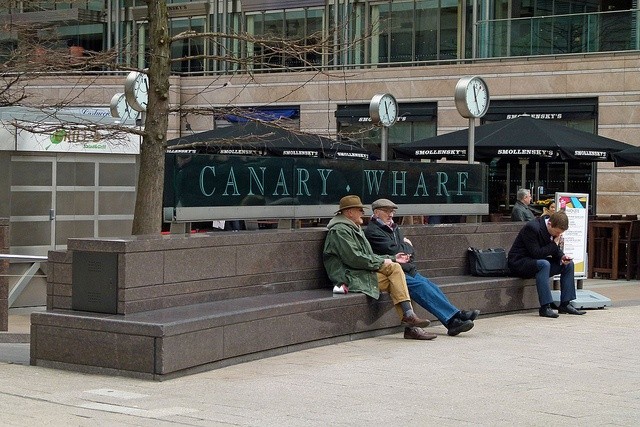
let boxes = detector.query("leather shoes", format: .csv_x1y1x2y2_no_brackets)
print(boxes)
448,319,474,336
401,313,430,328
558,304,586,315
404,326,437,339
539,305,559,318
458,311,478,321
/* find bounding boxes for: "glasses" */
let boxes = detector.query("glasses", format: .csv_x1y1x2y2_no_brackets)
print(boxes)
380,208,397,215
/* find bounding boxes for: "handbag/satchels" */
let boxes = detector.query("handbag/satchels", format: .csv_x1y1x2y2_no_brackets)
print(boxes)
467,246,509,277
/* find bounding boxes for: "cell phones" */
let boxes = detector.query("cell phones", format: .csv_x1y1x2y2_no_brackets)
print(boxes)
565,256,574,261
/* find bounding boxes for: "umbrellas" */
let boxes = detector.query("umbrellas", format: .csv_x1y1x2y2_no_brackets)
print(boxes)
166,119,369,159
393,114,637,187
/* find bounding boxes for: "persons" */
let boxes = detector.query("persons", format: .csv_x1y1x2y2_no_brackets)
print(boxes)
511,188,536,221
364,198,477,336
323,195,437,340
539,201,555,216
506,211,587,317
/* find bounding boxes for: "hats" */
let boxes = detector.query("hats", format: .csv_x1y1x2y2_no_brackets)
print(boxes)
372,199,398,210
334,195,369,214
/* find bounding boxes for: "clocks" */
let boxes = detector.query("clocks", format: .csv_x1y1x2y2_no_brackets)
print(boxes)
466,78,488,118
134,74,152,111
379,95,396,126
116,95,138,120
